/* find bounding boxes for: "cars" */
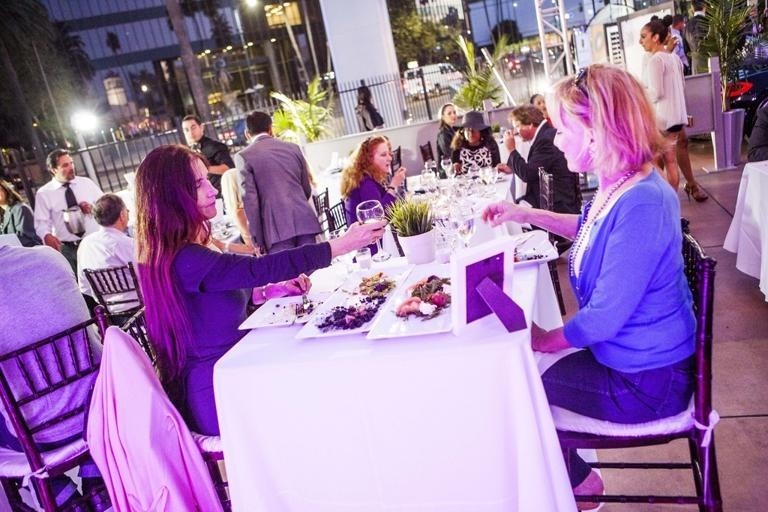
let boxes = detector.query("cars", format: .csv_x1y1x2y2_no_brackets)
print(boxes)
719,57,767,137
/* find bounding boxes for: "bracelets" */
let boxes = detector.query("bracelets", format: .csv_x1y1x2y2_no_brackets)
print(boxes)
224,241,230,253
387,186,396,192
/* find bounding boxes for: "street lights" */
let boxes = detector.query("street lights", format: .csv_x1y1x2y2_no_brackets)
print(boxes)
264,0,309,88
231,0,259,86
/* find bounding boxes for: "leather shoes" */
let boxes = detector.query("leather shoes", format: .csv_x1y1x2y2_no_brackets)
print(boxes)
60,483,113,512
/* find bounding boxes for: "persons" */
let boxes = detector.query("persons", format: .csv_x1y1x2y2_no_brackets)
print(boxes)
0,232,115,511
0,177,44,249
75,194,146,329
735,0,767,162
480,63,700,512
340,134,408,262
220,137,260,256
639,15,710,203
33,147,107,318
652,13,692,76
435,94,582,256
182,114,238,213
128,141,388,435
233,110,325,257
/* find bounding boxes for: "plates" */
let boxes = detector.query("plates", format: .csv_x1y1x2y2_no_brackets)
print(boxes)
238,293,319,328
365,265,453,340
495,230,558,265
292,266,412,341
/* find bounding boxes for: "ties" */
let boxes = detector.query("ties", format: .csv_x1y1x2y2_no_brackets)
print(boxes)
63,183,85,237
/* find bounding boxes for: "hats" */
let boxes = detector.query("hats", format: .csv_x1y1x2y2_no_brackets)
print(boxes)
452,110,493,137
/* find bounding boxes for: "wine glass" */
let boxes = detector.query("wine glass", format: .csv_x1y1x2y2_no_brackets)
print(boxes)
441,158,452,182
355,200,390,262
424,161,438,188
486,168,499,195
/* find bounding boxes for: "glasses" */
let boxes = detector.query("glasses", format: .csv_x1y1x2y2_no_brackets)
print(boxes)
575,66,590,100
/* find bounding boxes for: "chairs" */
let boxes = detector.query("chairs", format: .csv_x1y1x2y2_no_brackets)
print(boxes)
560,216,722,512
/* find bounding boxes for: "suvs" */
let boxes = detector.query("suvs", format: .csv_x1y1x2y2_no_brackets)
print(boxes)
403,62,463,100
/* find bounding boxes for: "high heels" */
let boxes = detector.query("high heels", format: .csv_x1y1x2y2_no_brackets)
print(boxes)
684,183,708,202
